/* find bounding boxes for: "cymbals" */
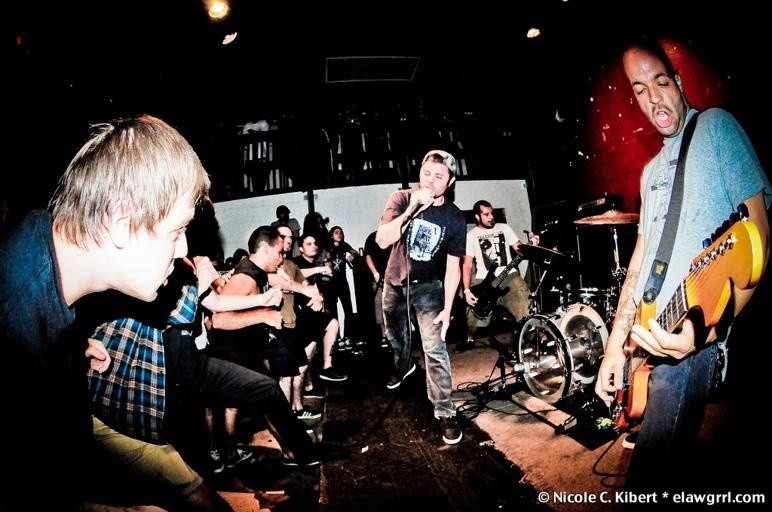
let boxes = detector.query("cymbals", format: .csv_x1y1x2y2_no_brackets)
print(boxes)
574,210,641,225
517,243,582,276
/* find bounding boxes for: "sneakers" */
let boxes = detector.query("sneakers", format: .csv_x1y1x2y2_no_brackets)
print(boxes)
337,339,346,352
226,449,253,467
439,416,463,445
303,389,324,398
319,368,347,381
345,336,353,350
297,409,321,420
209,448,224,474
387,363,416,389
456,337,476,350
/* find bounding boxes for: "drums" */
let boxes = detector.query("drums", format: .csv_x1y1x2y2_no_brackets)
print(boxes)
578,287,618,315
517,303,610,404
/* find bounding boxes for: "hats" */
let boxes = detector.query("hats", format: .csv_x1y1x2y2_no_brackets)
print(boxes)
421,150,456,175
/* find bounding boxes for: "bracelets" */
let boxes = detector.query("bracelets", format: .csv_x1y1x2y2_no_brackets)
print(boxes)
462,288,471,293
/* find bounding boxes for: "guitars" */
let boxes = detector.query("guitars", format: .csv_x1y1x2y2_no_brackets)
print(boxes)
609,203,765,434
464,220,560,327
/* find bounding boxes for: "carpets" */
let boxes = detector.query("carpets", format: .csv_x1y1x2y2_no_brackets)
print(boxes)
413,332,718,511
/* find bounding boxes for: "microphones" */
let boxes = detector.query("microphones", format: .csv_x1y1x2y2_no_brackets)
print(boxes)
411,197,435,219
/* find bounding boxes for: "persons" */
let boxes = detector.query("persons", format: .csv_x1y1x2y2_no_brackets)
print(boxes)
363,231,392,350
0,114,235,512
374,149,467,446
200,204,358,468
462,199,541,345
84,260,346,476
593,41,770,510
